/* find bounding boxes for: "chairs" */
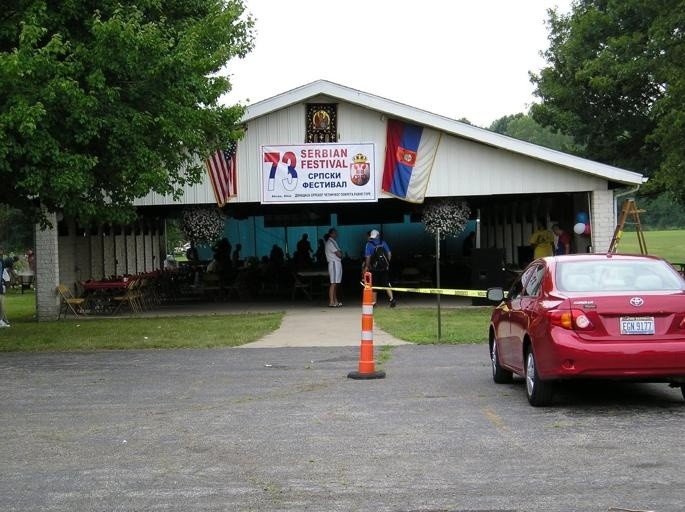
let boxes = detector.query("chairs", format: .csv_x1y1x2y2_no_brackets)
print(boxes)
54,261,209,323
200,261,433,303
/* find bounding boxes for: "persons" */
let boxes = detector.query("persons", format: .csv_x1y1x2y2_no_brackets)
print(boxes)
3,255,19,279
551,224,575,255
324,228,344,308
527,226,555,260
363,229,397,307
185,233,328,285
462,231,476,257
0,247,11,329
26,247,36,273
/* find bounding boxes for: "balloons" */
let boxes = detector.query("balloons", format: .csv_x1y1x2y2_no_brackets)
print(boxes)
576,212,588,224
584,224,591,235
573,222,586,234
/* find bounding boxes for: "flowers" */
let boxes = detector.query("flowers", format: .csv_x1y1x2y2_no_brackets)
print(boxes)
420,197,473,242
180,203,225,249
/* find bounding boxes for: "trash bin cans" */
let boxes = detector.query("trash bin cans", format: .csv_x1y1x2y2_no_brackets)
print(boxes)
517,246,533,270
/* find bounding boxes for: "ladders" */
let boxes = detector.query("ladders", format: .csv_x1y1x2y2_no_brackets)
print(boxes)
608,199,648,256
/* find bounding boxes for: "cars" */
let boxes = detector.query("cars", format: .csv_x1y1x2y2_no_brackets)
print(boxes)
483,250,685,409
173,241,191,257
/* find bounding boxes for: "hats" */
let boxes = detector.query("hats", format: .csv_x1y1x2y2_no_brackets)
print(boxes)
370,229,379,239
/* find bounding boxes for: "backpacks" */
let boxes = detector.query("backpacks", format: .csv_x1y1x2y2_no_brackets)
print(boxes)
369,240,390,272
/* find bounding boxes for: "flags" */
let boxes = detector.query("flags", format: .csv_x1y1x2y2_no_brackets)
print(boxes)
380,116,442,205
203,137,238,209
